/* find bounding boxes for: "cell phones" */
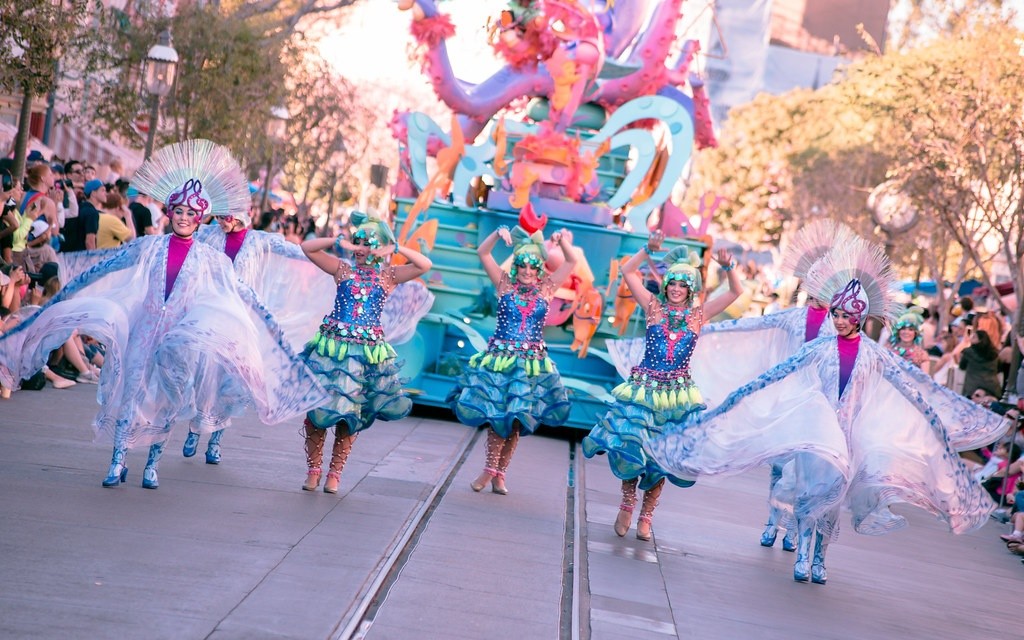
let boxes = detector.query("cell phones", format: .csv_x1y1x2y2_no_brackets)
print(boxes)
31,202,37,211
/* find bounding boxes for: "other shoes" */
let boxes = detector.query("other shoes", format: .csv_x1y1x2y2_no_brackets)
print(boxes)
760,523,798,552
182,432,221,464
53,377,76,388
75,363,101,384
470,476,508,494
793,561,827,584
614,512,651,541
990,512,1024,564
302,470,337,493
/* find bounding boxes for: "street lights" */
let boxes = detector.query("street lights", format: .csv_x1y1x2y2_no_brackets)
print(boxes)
261,99,291,209
142,27,179,164
324,130,347,237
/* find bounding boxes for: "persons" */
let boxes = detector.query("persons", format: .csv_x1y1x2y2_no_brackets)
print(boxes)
301,211,433,493
0,131,349,488
698,280,1024,583
582,232,743,539
446,225,578,495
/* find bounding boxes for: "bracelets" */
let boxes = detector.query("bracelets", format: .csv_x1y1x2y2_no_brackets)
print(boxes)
333,235,344,253
395,242,398,254
722,261,734,271
497,225,510,235
644,244,655,255
554,229,564,241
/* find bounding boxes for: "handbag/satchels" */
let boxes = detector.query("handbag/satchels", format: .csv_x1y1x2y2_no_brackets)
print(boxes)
20,366,47,391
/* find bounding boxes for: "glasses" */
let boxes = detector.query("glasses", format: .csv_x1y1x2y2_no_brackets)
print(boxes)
973,393,981,398
73,169,84,174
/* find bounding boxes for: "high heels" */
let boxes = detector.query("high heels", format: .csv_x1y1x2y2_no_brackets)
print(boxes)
102,467,128,486
142,469,159,488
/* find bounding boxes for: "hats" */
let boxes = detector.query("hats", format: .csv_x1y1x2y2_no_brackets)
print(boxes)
0,271,10,285
662,245,702,302
27,151,48,162
779,216,924,345
504,226,548,285
36,261,59,286
350,210,398,263
129,139,252,230
50,162,65,175
30,220,56,238
83,179,103,196
